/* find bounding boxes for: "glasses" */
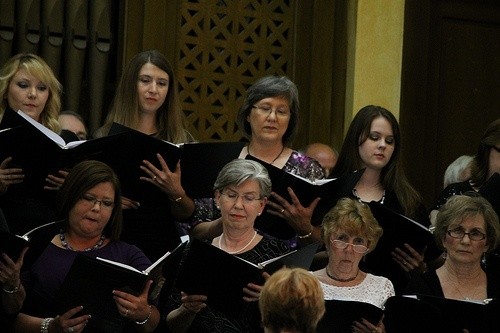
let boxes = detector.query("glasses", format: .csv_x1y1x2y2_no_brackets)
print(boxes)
79,193,115,209
249,104,292,116
219,187,263,202
329,239,370,254
492,145,500,153
444,228,488,241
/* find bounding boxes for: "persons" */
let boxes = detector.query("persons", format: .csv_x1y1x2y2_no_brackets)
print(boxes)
0,52,500,333
